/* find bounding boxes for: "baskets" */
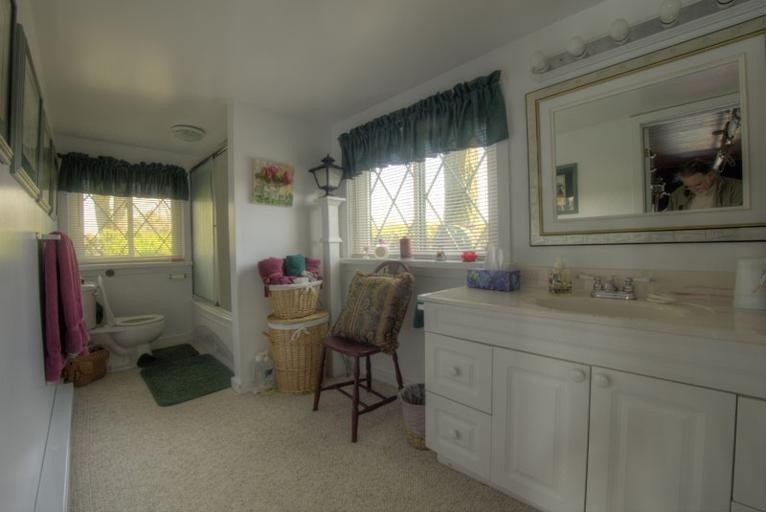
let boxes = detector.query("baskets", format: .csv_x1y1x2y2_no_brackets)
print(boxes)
266,280,330,394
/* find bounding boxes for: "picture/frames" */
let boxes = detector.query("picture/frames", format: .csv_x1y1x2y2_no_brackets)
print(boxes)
1,0,59,222
557,162,579,216
630,92,740,213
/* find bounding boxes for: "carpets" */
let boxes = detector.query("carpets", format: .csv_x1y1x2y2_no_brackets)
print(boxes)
139,353,235,406
136,343,200,367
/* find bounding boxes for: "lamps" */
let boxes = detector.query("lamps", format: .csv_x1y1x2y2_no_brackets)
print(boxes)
171,125,206,143
529,1,741,78
308,153,346,197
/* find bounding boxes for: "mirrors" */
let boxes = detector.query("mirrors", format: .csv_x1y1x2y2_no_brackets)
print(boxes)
525,12,766,246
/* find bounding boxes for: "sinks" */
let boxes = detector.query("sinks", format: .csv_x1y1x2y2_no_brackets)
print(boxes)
532,296,680,321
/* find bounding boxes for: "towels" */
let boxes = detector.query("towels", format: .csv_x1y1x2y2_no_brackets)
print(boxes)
42,230,91,385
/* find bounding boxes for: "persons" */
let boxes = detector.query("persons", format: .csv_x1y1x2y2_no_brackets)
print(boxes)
668,160,743,211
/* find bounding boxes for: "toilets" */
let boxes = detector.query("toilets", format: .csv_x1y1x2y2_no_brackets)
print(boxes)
79,274,166,374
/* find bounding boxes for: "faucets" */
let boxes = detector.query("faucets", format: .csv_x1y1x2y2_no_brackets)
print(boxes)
603,273,620,293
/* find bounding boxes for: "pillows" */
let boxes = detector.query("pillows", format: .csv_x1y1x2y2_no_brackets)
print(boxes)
329,270,416,355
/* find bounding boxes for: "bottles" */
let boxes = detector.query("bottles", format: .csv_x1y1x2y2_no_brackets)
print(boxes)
399,236,411,259
374,240,389,259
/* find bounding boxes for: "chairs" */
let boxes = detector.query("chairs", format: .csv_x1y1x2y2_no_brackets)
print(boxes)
313,259,410,443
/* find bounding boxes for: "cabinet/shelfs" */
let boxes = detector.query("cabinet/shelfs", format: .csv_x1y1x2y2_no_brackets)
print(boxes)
499,287,589,512
730,295,765,511
421,282,499,485
588,293,735,512
